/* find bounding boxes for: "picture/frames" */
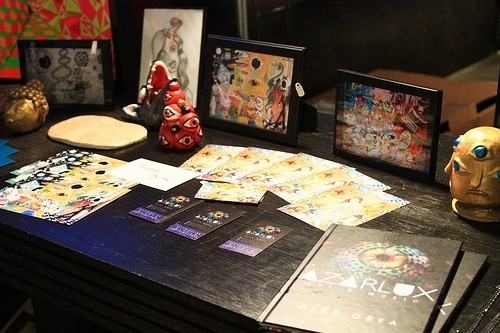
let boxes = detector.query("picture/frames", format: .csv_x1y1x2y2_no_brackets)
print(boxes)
17,39,115,111
199,34,306,147
333,70,443,189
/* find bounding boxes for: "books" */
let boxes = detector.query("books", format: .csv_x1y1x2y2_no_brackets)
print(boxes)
430,249,490,333
255,223,463,333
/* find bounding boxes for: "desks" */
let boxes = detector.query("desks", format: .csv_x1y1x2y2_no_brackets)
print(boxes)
0,102,500,333
